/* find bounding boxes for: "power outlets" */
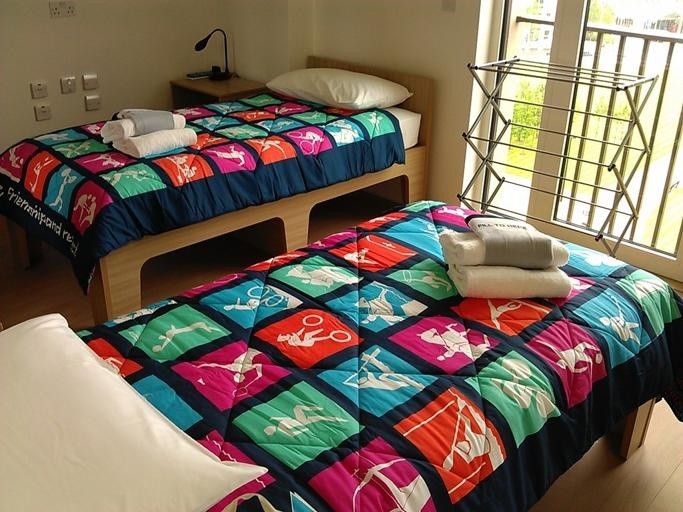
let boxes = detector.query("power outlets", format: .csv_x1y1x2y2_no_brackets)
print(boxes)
49,1,78,18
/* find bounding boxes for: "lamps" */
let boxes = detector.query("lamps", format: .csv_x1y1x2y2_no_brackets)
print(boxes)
194,28,232,80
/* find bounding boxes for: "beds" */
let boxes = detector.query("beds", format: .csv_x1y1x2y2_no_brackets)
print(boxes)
0,55,434,318
4,199,683,512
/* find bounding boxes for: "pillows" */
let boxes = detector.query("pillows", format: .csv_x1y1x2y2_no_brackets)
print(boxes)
1,313,270,512
265,67,413,112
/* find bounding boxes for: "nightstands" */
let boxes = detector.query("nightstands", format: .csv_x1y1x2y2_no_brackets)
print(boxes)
169,71,264,109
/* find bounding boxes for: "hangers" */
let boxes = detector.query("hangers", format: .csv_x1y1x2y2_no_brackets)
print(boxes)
455,56,660,260
